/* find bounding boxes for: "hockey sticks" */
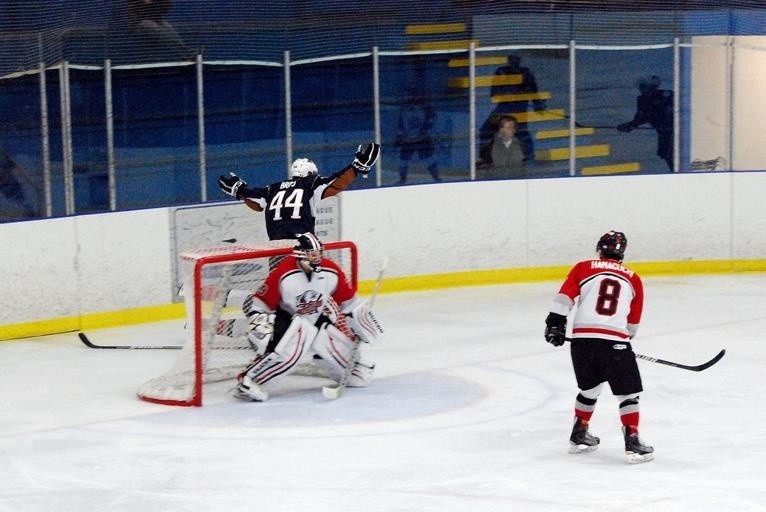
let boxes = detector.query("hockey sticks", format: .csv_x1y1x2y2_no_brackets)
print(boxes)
78,333,255,351
545,324,726,372
323,258,390,399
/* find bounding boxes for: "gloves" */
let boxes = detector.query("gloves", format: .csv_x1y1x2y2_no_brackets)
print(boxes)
218,172,247,200
352,142,380,174
544,312,567,346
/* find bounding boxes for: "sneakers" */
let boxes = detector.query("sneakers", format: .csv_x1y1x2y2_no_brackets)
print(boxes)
570,430,599,446
625,436,653,455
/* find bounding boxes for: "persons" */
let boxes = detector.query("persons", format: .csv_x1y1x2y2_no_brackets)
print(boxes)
236,232,376,402
480,116,534,169
544,230,654,455
476,54,545,170
217,142,381,354
396,86,441,186
617,74,674,173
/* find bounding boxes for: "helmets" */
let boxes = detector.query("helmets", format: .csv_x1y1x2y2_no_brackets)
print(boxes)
291,157,318,177
293,232,323,273
596,230,627,259
